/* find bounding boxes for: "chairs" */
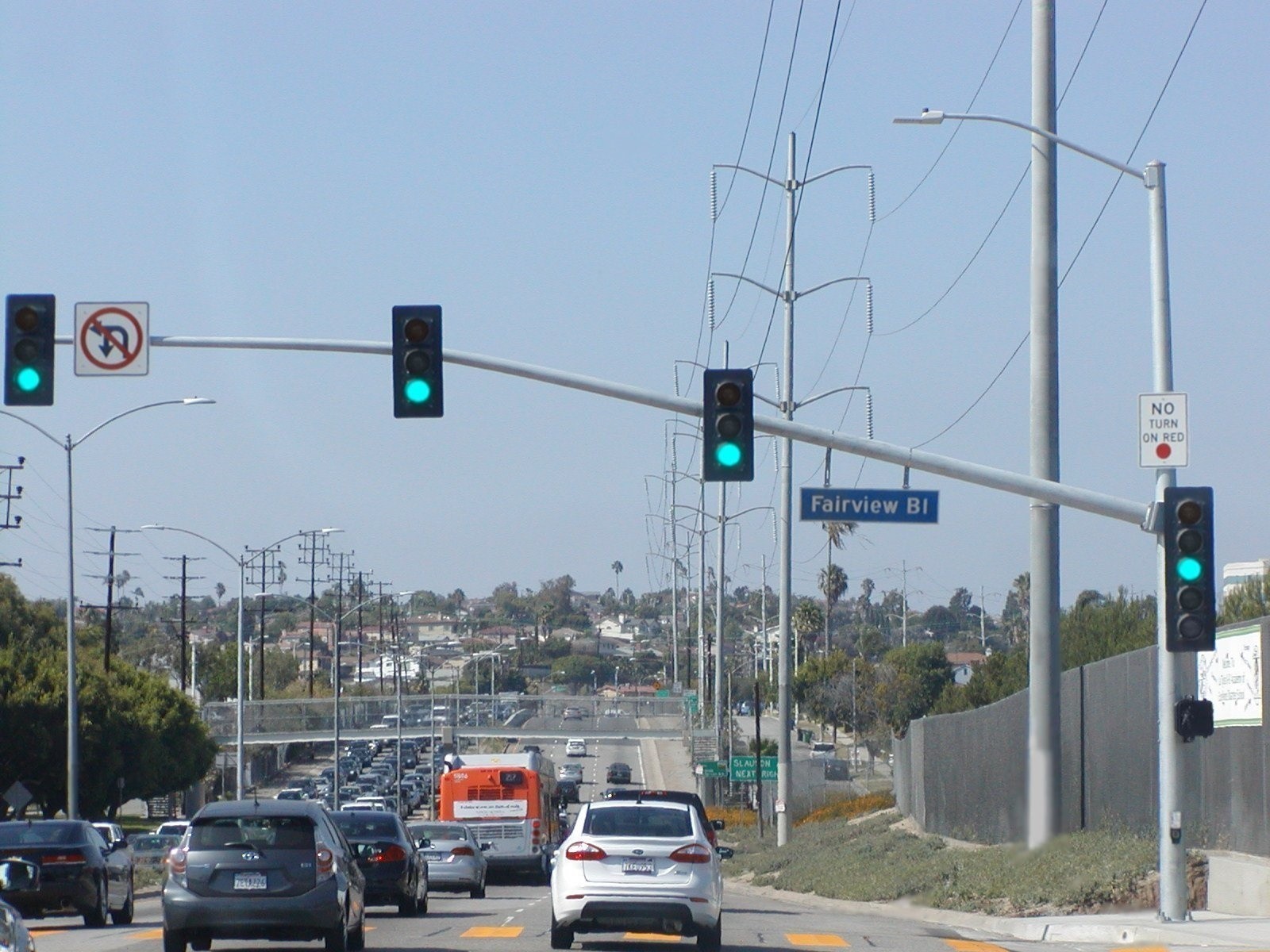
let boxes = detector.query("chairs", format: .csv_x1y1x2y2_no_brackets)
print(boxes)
208,821,242,846
442,830,465,841
413,829,425,839
591,813,616,834
274,822,310,848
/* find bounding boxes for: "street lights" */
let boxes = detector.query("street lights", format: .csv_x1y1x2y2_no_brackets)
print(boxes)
389,645,502,821
337,641,465,814
889,110,1194,924
140,524,347,830
727,656,774,799
413,652,501,755
458,636,532,729
1,396,217,819
254,591,418,811
436,645,519,755
541,671,566,697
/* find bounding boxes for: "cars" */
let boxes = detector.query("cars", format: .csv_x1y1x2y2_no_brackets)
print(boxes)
92,821,136,926
808,744,838,766
159,800,376,952
541,800,735,952
736,699,763,717
0,858,40,952
275,704,633,918
0,820,130,928
560,788,725,833
823,760,849,781
125,820,191,872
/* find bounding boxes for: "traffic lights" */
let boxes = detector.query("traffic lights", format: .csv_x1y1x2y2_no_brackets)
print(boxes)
391,304,445,419
703,368,755,483
3,293,56,407
1164,486,1216,653
1174,698,1214,738
